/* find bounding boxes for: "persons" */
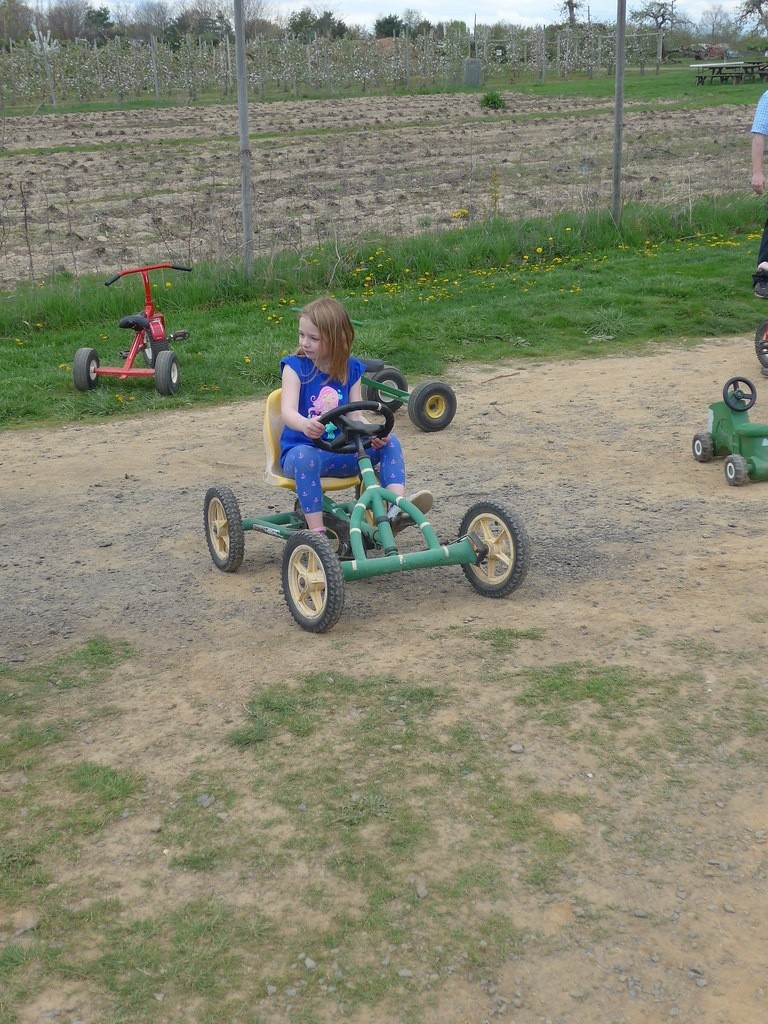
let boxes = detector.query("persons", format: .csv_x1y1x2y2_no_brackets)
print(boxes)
750,90,768,298
280,297,434,543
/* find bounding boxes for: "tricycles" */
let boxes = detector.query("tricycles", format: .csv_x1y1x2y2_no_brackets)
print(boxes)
287,307,457,433
72,263,192,396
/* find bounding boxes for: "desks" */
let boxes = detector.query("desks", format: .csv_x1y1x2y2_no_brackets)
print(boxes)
689,60,768,85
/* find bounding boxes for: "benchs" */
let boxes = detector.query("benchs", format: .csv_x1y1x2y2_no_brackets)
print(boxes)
696,69,768,78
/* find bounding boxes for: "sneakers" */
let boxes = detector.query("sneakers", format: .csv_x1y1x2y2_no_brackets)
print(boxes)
385,489,434,532
312,527,330,542
753,269,768,299
